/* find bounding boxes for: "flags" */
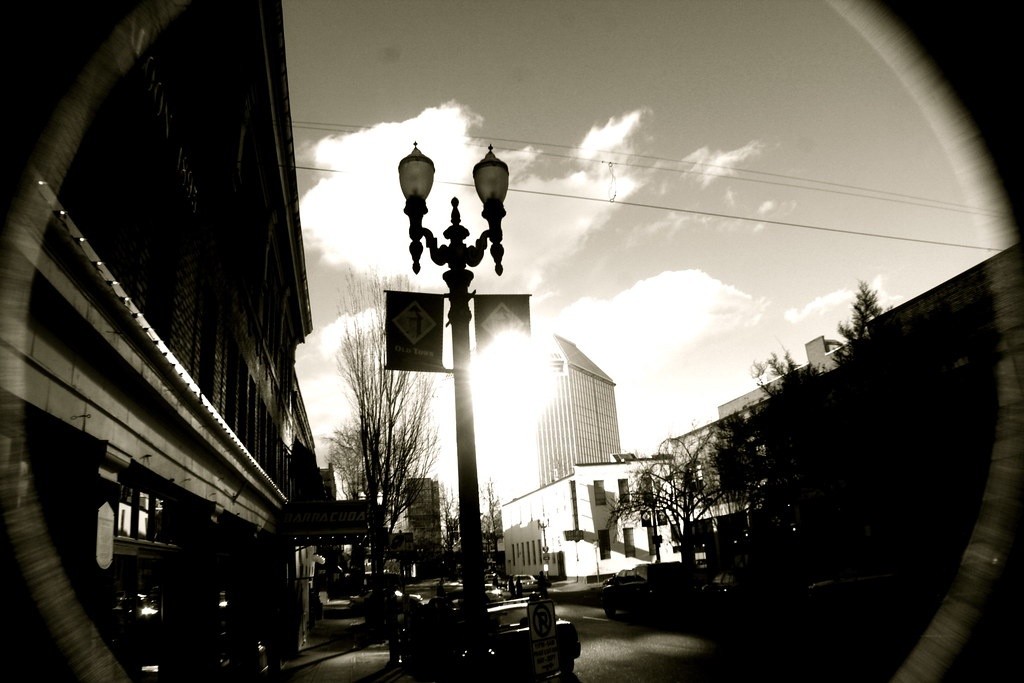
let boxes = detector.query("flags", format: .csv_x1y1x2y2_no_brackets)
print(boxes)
473,292,534,339
383,291,448,375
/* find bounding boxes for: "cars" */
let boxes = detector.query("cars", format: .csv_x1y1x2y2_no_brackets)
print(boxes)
507,574,540,593
484,583,504,602
483,603,581,675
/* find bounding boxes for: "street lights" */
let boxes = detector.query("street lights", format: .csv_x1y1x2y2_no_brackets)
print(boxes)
397,143,510,683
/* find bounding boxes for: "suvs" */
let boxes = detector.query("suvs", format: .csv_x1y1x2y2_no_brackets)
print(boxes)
602,562,686,619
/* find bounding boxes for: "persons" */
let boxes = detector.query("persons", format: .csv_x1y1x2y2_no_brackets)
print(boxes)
537,571,549,598
509,575,523,600
